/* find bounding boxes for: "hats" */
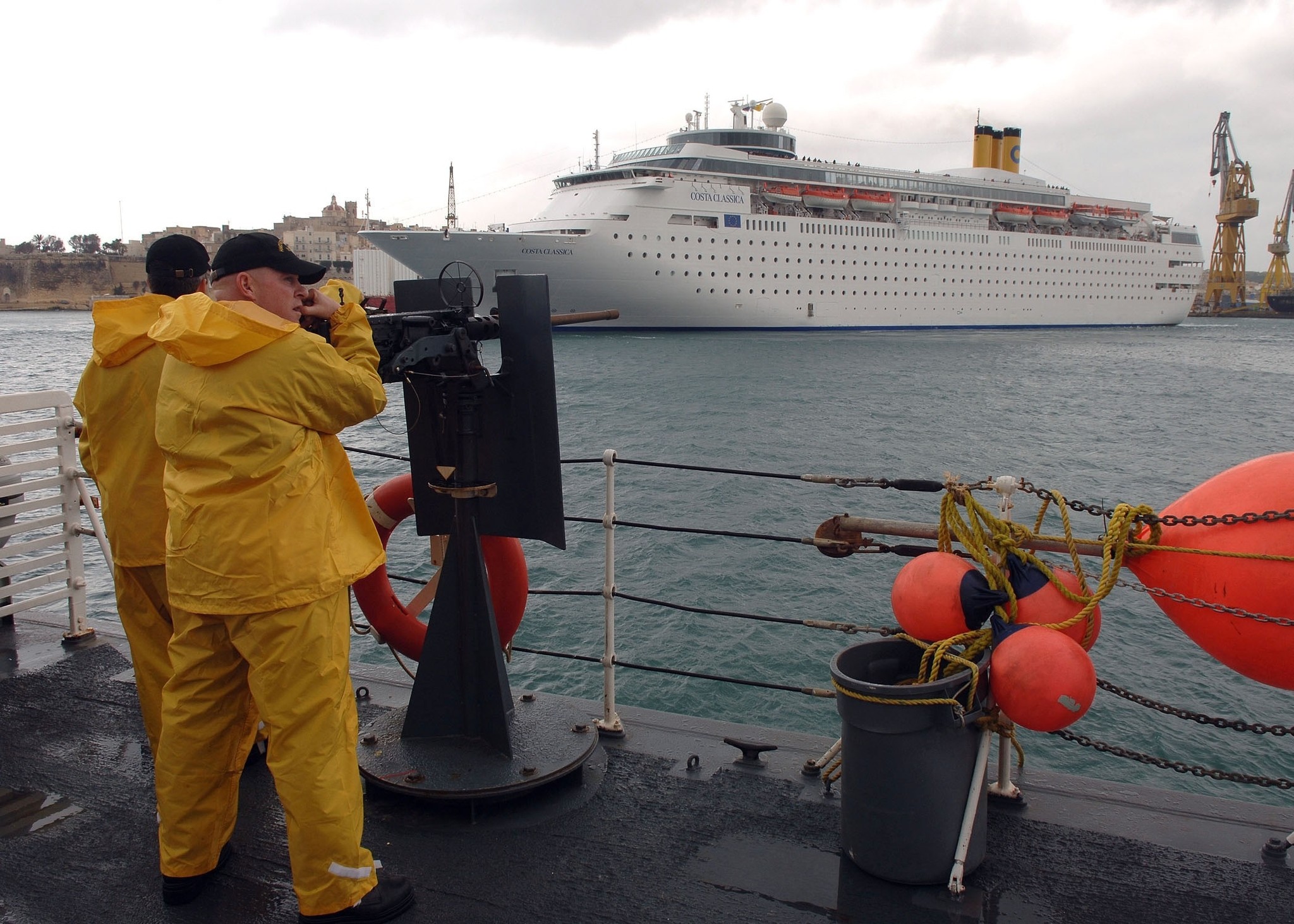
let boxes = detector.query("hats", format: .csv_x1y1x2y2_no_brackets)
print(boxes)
146,234,212,279
208,231,328,285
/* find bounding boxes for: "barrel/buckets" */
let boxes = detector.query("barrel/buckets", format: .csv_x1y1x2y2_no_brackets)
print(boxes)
829,638,991,886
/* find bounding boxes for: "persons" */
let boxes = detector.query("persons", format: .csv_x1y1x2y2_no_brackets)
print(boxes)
70,234,269,774
151,232,417,924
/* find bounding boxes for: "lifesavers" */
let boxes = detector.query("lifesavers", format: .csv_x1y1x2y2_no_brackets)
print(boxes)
351,473,529,663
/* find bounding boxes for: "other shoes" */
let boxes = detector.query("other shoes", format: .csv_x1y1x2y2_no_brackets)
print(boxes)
161,841,233,903
298,877,416,924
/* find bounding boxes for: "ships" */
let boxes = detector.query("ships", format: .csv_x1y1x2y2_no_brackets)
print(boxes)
355,93,1203,328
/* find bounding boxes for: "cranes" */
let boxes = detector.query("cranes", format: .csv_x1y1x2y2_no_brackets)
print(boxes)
1199,112,1260,308
1258,168,1294,308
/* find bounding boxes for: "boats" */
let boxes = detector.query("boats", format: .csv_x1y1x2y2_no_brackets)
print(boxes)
1031,210,1069,227
800,187,849,211
1100,209,1141,228
993,207,1033,224
1068,207,1109,225
761,186,802,205
849,190,895,213
